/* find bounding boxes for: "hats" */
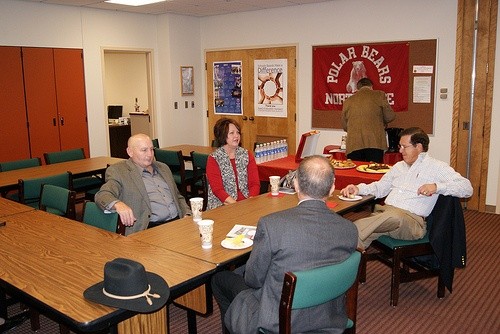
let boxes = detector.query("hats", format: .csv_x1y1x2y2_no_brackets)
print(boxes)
83,258,170,313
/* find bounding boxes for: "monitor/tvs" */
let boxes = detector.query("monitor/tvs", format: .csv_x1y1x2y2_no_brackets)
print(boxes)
107,106,123,123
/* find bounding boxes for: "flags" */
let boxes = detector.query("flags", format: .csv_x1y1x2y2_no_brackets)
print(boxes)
313,43,410,112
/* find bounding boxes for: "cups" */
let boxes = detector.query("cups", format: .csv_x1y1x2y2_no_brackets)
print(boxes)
196,220,214,249
269,176,281,195
189,198,203,222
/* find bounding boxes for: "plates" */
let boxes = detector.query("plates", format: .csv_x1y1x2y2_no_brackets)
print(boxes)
356,165,392,173
220,238,253,249
339,196,362,202
334,165,356,169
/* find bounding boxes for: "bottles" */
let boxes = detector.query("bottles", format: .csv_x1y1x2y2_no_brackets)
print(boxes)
255,139,288,164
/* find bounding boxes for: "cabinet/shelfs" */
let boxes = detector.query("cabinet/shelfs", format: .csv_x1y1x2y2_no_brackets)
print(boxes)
0,44,92,164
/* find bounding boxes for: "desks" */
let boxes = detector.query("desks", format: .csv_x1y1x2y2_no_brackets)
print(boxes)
322,145,404,166
156,143,220,161
0,156,375,334
253,155,400,191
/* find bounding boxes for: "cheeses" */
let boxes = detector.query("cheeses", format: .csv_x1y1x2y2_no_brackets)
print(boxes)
233,234,244,245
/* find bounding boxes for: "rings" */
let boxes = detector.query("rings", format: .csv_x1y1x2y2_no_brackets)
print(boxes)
424,192,426,194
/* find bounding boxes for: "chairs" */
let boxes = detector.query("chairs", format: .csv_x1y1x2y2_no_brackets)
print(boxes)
151,139,160,148
153,148,196,211
0,158,40,173
190,150,209,212
81,199,121,233
256,250,364,334
17,170,84,204
37,183,79,218
369,191,477,310
43,148,86,165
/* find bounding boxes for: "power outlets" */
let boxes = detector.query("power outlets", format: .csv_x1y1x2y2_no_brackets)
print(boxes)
173,101,195,110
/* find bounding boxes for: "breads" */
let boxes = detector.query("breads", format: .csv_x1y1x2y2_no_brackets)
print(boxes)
329,159,354,167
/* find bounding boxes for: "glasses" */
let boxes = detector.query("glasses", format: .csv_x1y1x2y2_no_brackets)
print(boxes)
398,143,417,149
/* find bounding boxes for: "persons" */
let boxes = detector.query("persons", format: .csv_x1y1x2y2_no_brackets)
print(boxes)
95,133,193,236
341,78,396,163
206,118,260,210
341,127,474,250
212,155,358,334
